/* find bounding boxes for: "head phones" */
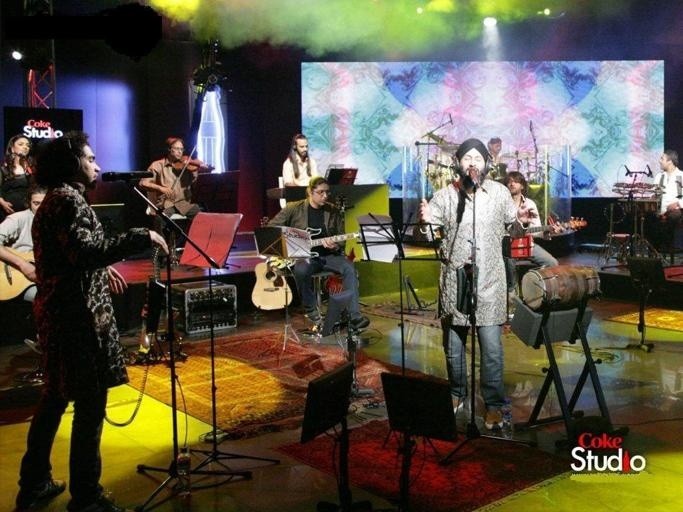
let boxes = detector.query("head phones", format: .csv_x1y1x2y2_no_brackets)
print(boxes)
452,155,496,172
61,138,81,176
292,141,297,150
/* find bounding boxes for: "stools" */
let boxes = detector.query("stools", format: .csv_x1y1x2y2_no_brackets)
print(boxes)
651,219,683,265
292,270,337,339
145,204,189,268
501,258,537,326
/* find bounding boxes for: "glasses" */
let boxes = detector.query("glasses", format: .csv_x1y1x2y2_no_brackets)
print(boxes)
171,146,185,152
313,189,331,196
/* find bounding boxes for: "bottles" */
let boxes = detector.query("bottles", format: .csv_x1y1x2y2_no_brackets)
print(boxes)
177,447,193,500
500,399,514,438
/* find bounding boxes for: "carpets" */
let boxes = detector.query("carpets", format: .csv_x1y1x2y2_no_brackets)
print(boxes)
360,297,512,336
121,321,455,441
604,304,683,334
272,419,572,511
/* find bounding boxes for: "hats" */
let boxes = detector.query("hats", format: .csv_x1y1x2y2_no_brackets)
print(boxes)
455,139,489,164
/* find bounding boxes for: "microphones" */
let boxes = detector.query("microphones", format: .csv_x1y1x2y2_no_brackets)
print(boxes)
466,167,481,188
646,164,655,178
624,165,633,177
530,119,533,131
101,167,155,183
324,201,346,217
448,113,453,125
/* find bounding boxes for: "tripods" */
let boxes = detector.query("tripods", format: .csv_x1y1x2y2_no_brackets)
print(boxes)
278,278,300,351
440,195,536,463
316,416,371,512
187,272,280,473
626,282,655,354
137,255,253,509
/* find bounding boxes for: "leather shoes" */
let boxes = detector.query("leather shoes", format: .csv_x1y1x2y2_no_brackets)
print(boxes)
305,307,320,321
348,317,369,330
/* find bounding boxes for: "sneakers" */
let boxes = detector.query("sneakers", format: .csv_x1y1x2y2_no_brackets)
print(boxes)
451,394,464,413
67,490,130,512
16,480,66,511
485,410,503,430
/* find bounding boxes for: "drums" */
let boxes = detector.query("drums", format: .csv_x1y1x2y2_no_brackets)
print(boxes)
522,265,601,309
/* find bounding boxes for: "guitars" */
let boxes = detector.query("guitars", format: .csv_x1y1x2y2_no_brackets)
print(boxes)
271,227,362,267
252,217,293,311
504,217,586,239
0,246,37,300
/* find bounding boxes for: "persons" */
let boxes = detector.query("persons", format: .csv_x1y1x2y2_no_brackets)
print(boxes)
266,174,372,338
0,183,50,355
485,137,504,170
282,132,321,187
649,148,683,224
13,130,170,510
137,137,210,267
417,138,539,431
0,135,39,219
502,171,559,320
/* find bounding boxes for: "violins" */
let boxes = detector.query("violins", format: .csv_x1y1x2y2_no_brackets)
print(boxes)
173,155,215,172
